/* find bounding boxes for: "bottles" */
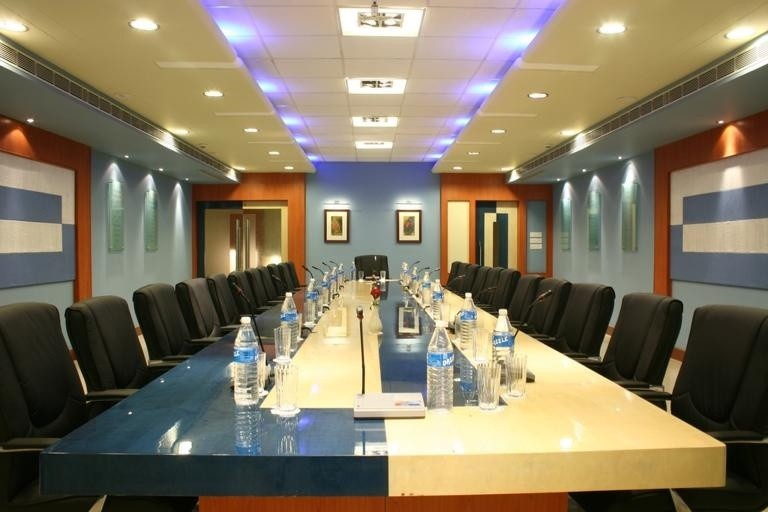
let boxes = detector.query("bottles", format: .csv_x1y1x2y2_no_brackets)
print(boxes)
231,315,260,406
491,309,515,377
400,261,443,320
275,417,299,454
280,291,298,353
424,319,456,413
305,263,345,322
233,407,261,454
459,292,478,351
459,353,478,402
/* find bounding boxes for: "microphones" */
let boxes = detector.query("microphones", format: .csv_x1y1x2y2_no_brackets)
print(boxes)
448,286,498,331
329,260,349,283
498,289,553,384
424,275,465,310
399,261,421,283
270,273,313,333
230,280,268,367
403,266,430,291
312,265,339,299
321,261,344,290
415,268,441,297
302,265,330,313
357,307,366,394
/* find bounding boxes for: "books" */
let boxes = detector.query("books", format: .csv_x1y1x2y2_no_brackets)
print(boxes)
352,392,426,419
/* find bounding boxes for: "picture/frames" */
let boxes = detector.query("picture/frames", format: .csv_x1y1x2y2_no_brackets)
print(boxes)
393,209,424,245
322,208,350,244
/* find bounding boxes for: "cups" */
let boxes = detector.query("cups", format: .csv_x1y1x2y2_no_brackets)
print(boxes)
359,271,364,282
295,312,303,340
258,351,268,396
477,363,501,407
272,327,292,360
439,303,451,321
271,358,301,411
505,351,530,399
378,270,387,284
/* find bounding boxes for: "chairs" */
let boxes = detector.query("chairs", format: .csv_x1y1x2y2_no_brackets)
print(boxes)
567,302,767,510
583,290,684,411
66,298,170,407
130,259,302,363
0,300,198,511
441,260,615,361
353,254,389,281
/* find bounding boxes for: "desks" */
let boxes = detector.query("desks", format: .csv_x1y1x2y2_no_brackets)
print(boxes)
39,275,729,512
